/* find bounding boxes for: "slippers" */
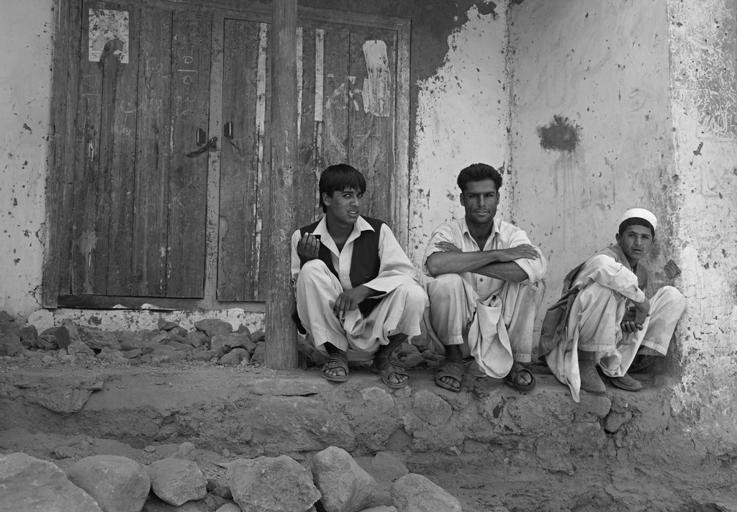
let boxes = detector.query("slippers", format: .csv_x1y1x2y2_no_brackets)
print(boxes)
503,362,535,392
434,357,464,392
373,355,410,390
321,353,350,382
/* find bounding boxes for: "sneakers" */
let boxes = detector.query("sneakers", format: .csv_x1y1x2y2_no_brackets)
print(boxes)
581,362,607,394
607,373,643,391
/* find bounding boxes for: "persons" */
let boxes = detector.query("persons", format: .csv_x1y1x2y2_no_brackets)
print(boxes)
538,206,684,403
420,163,546,393
288,163,428,390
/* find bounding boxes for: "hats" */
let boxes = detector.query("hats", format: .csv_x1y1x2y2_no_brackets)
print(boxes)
618,207,658,232
